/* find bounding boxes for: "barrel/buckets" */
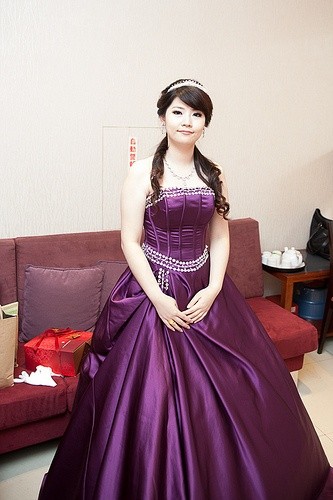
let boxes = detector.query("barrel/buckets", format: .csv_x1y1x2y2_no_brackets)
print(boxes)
298,288,328,320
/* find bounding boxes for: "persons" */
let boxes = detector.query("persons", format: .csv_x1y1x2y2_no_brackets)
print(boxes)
38,79,333,500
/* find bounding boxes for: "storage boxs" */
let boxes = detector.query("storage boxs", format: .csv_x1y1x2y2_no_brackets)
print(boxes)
24,329,94,378
265,295,299,318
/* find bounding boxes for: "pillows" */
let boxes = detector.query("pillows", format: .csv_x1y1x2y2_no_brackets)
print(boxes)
17,263,104,343
98,260,128,320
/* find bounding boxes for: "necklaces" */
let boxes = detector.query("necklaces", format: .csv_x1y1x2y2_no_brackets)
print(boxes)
163,155,196,180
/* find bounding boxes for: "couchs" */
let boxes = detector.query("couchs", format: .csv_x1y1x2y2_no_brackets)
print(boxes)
0,218,319,456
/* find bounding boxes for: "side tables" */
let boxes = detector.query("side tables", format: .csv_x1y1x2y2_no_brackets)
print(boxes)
261,249,333,339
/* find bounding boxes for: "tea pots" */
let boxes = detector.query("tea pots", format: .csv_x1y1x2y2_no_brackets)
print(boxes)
281,246,304,267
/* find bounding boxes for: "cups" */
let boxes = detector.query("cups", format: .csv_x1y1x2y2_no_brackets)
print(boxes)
261,250,282,267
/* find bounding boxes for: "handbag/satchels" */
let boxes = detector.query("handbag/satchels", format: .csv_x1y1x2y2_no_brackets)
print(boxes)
0,301,18,389
306,208,333,261
